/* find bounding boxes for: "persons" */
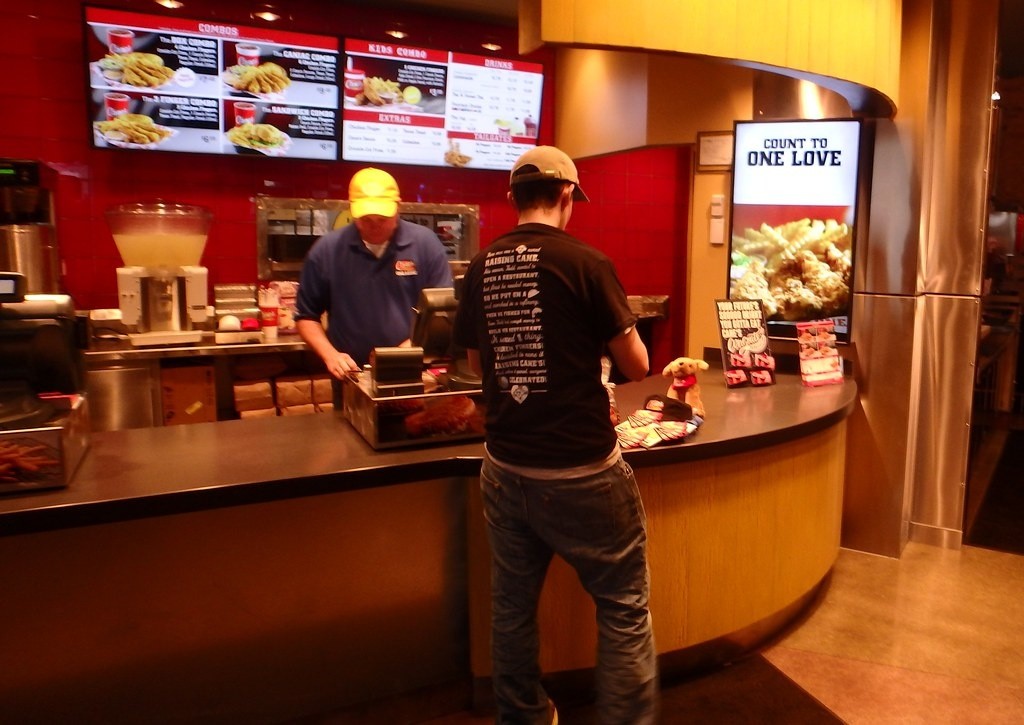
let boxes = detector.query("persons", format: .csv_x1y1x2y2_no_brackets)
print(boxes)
453,146,655,725
292,166,454,412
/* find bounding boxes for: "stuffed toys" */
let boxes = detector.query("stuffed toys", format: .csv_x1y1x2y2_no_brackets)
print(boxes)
662,357,709,419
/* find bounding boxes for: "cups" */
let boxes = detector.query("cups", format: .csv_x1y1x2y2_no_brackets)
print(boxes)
235,43,261,67
234,102,257,128
103,93,131,121
106,28,135,56
344,68,366,97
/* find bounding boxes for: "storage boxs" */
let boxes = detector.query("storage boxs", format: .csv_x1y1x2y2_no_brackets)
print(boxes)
1,394,90,493
162,365,218,427
233,374,335,419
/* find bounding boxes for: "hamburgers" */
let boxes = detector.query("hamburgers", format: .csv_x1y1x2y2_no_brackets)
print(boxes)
249,124,281,147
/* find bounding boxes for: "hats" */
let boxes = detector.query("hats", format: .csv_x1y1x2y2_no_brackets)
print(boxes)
348,167,401,219
510,146,590,202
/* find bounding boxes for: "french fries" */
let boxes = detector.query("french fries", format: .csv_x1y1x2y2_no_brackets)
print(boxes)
729,218,848,273
